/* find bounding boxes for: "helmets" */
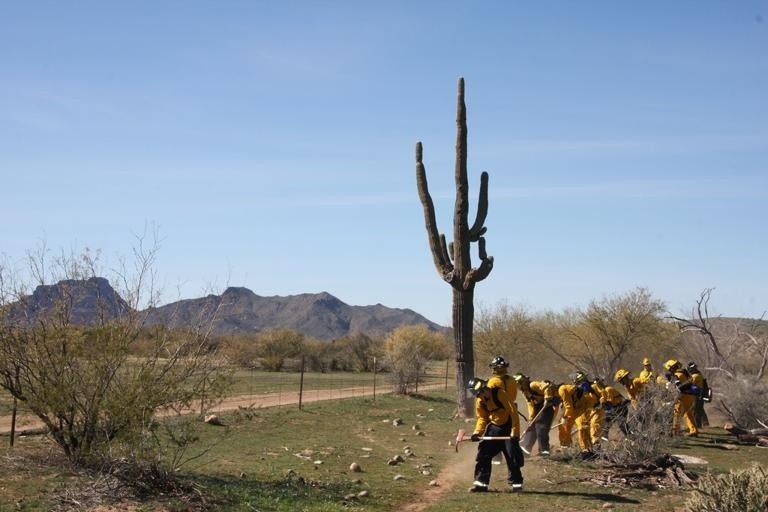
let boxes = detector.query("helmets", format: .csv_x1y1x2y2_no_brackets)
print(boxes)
541,380,555,388
614,369,631,383
513,372,529,386
665,359,678,371
687,361,698,373
488,356,509,370
640,358,652,366
573,370,588,384
468,377,489,398
593,377,604,386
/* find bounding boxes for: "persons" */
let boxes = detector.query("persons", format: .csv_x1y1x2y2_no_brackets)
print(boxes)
468,378,523,492
512,373,554,457
542,380,591,453
571,356,712,445
486,357,520,465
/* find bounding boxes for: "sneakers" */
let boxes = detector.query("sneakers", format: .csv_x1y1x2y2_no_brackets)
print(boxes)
505,486,522,493
468,485,489,492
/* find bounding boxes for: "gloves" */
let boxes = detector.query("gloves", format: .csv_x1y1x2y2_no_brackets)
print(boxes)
560,417,568,424
508,435,520,443
471,434,480,441
543,400,553,409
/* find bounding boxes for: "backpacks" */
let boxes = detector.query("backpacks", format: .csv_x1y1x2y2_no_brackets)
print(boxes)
699,377,712,403
